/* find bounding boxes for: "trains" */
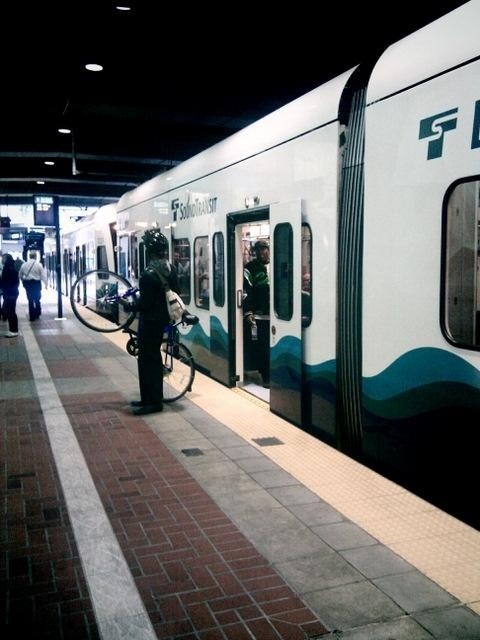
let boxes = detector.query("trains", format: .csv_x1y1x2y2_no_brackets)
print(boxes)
32,1,480,523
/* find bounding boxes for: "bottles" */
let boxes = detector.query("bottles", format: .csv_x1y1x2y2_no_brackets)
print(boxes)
251,322,258,340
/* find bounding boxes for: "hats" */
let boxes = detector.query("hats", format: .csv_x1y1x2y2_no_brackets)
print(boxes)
252,240,270,248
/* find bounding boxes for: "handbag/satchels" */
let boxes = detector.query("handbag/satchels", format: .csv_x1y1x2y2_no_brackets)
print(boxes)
165,290,185,321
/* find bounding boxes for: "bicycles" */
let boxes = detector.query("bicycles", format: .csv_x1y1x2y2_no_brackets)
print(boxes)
64,267,198,406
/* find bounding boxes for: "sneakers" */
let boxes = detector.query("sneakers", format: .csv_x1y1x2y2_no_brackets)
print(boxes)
4,332,19,337
131,401,163,415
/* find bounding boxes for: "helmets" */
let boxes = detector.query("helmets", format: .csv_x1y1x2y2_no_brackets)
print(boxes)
142,230,169,255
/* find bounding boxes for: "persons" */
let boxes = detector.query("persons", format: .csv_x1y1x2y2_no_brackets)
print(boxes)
244,241,270,388
118,231,177,415
0,253,48,338
